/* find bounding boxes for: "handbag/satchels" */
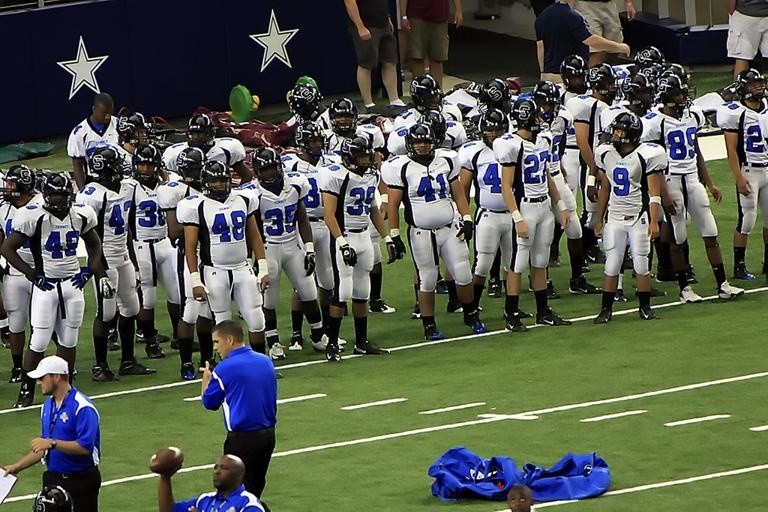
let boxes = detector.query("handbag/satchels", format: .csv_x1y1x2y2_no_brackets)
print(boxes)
427,446,522,504
522,451,610,502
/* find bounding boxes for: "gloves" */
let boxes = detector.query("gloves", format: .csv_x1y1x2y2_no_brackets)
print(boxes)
71,267,94,289
304,252,316,277
98,276,116,300
386,235,406,264
456,221,473,241
339,243,358,267
31,270,54,292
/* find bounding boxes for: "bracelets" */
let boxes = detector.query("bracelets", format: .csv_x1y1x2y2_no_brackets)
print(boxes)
50,438,58,448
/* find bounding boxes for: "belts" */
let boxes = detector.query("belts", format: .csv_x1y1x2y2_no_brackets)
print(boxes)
308,216,324,222
524,196,547,203
343,227,367,234
550,171,559,177
564,145,579,150
743,163,768,168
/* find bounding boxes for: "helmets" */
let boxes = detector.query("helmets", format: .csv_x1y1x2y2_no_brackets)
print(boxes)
559,45,698,111
41,172,74,213
1,165,36,203
252,148,284,191
477,78,561,151
199,160,232,200
734,68,767,103
603,112,644,146
340,136,374,176
404,110,448,161
295,121,328,160
131,144,162,184
409,74,443,113
176,147,206,185
184,114,215,151
89,145,126,184
290,84,323,121
328,97,359,137
115,111,153,148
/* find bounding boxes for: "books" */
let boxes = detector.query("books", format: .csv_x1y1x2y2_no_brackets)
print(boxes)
0,467,18,505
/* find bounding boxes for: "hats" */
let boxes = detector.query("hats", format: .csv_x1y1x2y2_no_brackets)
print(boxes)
26,356,69,380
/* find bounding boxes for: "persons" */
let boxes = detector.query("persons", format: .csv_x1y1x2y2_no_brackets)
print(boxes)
1,0,767,408
0,354,100,511
198,320,278,501
505,483,535,512
158,452,269,512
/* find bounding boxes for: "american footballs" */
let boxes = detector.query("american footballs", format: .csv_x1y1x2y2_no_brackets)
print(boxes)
148,445,184,472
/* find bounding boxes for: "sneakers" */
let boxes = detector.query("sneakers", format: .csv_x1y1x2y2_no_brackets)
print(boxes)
144,340,166,359
581,247,606,273
638,304,657,321
594,307,612,325
679,286,703,304
180,361,196,381
613,288,629,303
15,382,36,409
368,300,397,314
107,328,121,352
424,326,445,341
325,342,342,363
568,273,602,296
10,367,24,384
505,314,529,334
463,316,487,334
135,328,171,342
435,281,449,295
487,280,503,298
118,357,157,376
528,275,561,301
352,341,391,356
503,306,534,320
715,279,745,300
309,333,347,354
90,364,120,383
197,353,219,374
446,301,483,314
170,338,201,353
631,285,667,297
268,342,287,361
733,264,755,281
410,304,422,320
288,336,304,352
656,264,696,285
536,310,572,327
